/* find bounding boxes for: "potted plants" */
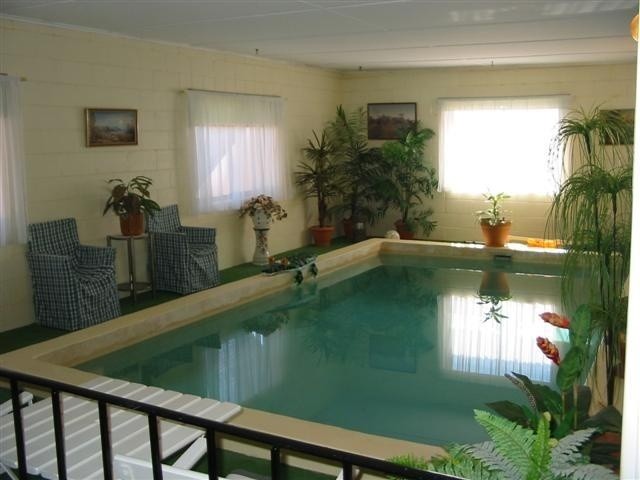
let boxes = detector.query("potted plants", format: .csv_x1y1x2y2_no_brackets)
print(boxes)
294,128,338,246
547,102,637,473
474,190,514,248
103,176,161,236
477,270,512,324
328,107,374,240
367,120,438,239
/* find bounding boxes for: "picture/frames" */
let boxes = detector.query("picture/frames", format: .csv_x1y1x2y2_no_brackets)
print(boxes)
251,210,272,266
597,107,635,146
83,107,138,149
363,99,420,144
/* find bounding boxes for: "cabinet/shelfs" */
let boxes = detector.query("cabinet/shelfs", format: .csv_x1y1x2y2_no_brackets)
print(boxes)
106,234,153,306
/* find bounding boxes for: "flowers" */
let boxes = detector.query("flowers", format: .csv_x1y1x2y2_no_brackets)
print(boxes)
239,193,287,222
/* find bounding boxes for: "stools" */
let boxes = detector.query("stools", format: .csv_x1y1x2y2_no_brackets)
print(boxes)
0,377,243,480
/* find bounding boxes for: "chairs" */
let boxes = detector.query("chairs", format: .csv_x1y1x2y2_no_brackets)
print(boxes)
113,450,365,480
24,218,121,332
145,204,220,294
